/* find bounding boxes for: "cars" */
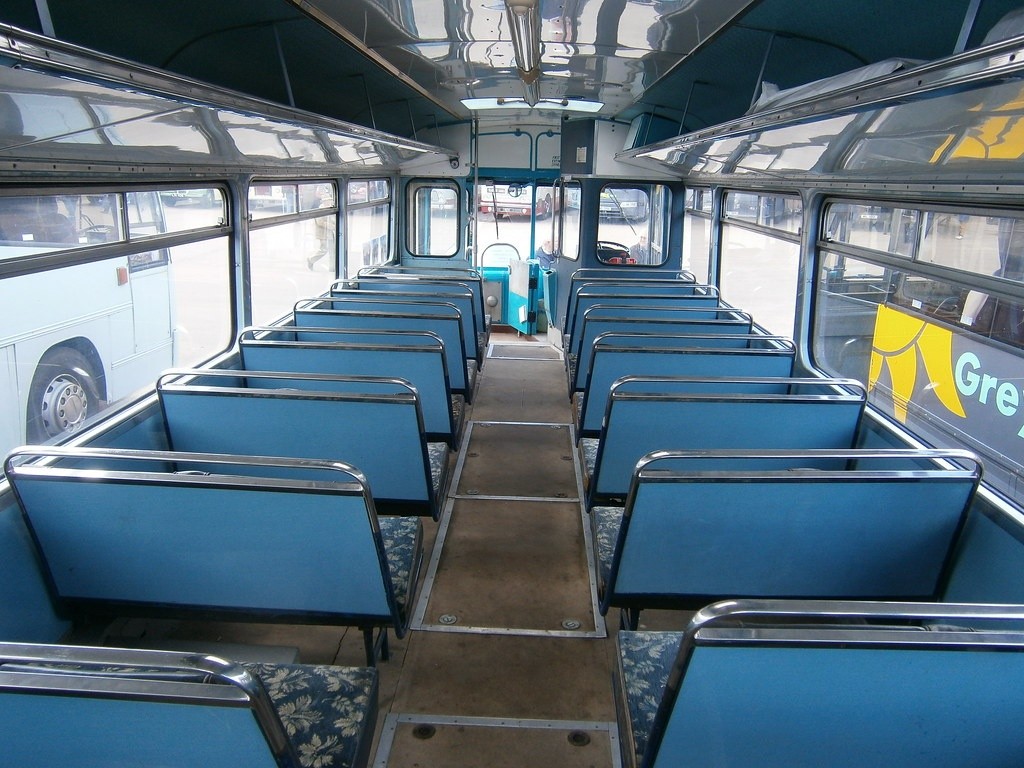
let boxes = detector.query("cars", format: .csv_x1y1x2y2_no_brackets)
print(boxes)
600,188,649,222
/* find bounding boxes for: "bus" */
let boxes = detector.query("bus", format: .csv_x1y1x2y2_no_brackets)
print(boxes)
481,185,567,220
0,191,180,479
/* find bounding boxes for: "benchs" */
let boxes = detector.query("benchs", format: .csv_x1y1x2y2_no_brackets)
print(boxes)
561,268,1024,768
0,266,492,767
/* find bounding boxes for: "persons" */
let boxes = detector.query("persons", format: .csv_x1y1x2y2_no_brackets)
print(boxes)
955,214,969,239
306,194,337,272
629,234,648,264
529,240,558,269
